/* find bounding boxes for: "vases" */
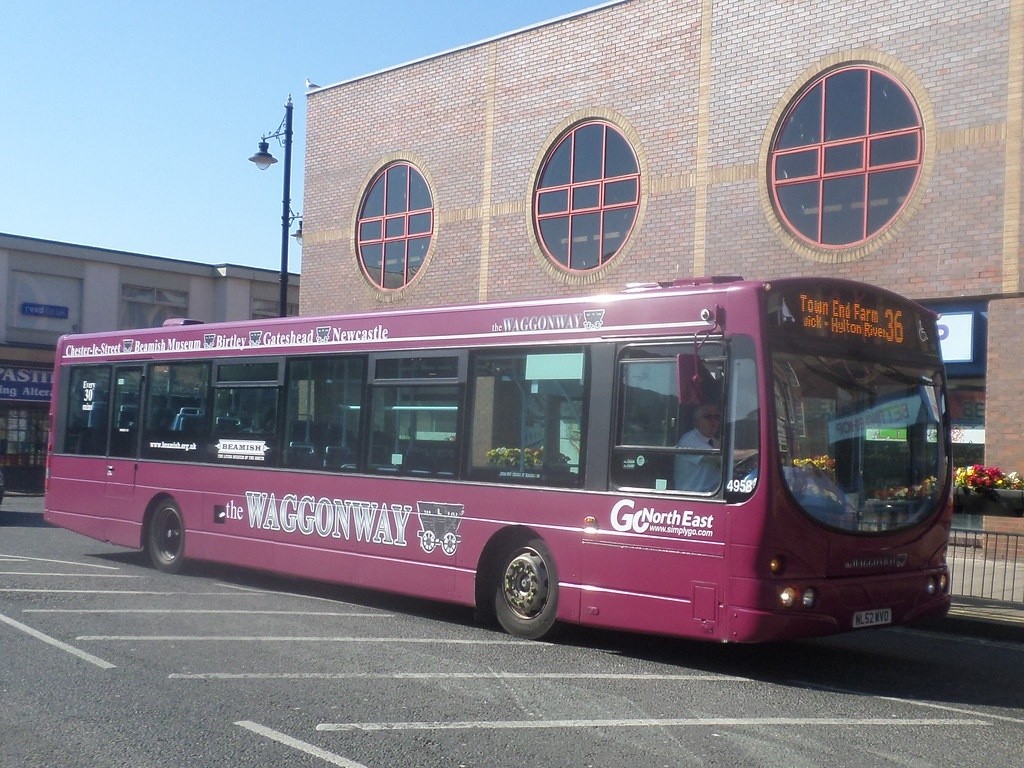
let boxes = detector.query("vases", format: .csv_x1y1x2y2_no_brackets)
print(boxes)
952,483,1024,518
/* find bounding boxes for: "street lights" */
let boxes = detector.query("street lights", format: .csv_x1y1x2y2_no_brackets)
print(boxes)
248,92,303,317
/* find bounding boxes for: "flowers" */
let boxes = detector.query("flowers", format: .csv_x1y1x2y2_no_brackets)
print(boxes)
875,464,1024,501
793,453,836,477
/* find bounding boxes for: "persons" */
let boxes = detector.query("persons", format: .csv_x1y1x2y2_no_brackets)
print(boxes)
673,403,758,493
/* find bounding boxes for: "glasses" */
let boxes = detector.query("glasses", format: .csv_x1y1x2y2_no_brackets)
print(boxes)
703,413,720,420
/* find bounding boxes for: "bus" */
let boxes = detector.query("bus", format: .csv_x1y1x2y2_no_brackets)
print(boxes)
42,277,953,643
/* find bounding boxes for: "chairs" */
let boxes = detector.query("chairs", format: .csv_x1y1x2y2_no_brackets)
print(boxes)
74,385,578,480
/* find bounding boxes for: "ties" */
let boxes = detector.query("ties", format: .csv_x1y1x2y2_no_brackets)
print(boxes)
707,439,714,446
